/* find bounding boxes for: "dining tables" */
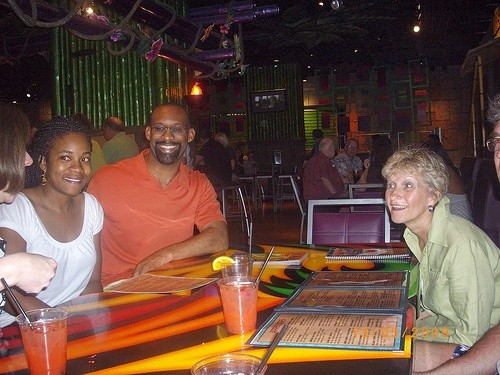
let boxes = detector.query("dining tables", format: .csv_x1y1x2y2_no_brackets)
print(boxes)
0,242,420,375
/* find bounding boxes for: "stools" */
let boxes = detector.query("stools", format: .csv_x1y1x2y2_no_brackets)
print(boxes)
211,162,296,245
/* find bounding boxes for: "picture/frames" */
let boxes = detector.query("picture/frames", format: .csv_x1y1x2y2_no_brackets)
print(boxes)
251,91,288,113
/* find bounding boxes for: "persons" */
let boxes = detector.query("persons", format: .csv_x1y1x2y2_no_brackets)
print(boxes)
302,130,390,212
87,103,229,287
427,140,473,221
0,117,105,326
202,130,246,208
412,98,500,375
90,117,139,172
0,104,58,311
382,147,500,347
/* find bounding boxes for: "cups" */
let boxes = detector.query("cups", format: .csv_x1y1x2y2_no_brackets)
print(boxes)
191,353,268,375
16,308,71,375
222,254,253,278
217,276,260,334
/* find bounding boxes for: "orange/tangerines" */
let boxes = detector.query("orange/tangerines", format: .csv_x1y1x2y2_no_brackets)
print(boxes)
212,255,234,271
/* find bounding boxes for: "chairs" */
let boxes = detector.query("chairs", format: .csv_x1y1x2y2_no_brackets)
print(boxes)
307,199,391,244
348,184,403,244
290,175,307,245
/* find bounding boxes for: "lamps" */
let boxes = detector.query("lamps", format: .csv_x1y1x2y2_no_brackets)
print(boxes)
186,96,209,106
412,14,422,32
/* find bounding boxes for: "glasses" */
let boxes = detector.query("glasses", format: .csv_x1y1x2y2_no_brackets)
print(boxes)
153,124,186,136
348,145,357,150
486,138,500,152
